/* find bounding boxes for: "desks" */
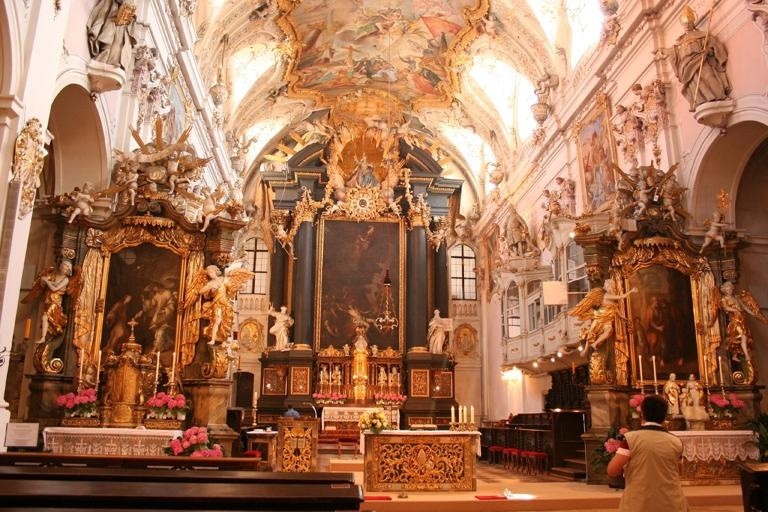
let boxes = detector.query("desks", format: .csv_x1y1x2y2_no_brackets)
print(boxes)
245,430,279,472
0,453,364,512
359,429,482,492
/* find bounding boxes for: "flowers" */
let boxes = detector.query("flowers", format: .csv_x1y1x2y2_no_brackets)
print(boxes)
705,392,744,416
55,387,188,419
310,389,408,434
591,425,631,465
163,422,226,457
629,394,644,413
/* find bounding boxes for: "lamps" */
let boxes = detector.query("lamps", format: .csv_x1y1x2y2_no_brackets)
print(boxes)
210,37,231,106
531,74,559,128
374,26,401,337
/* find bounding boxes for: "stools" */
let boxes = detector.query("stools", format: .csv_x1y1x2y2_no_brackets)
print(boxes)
487,446,549,476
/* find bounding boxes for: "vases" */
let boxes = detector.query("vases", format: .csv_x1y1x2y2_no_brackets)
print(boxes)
61,417,183,429
711,416,737,430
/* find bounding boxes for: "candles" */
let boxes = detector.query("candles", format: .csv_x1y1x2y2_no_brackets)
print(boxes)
702,354,724,385
637,351,657,381
155,349,176,382
450,404,475,422
78,347,102,381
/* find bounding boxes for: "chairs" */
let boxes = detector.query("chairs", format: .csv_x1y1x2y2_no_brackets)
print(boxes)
317,429,360,459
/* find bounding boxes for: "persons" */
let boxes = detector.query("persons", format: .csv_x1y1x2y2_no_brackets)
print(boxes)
316,363,404,388
19,151,256,355
88,1,137,72
266,302,296,349
281,403,301,417
422,309,448,354
606,394,691,511
668,6,732,109
500,80,768,415
